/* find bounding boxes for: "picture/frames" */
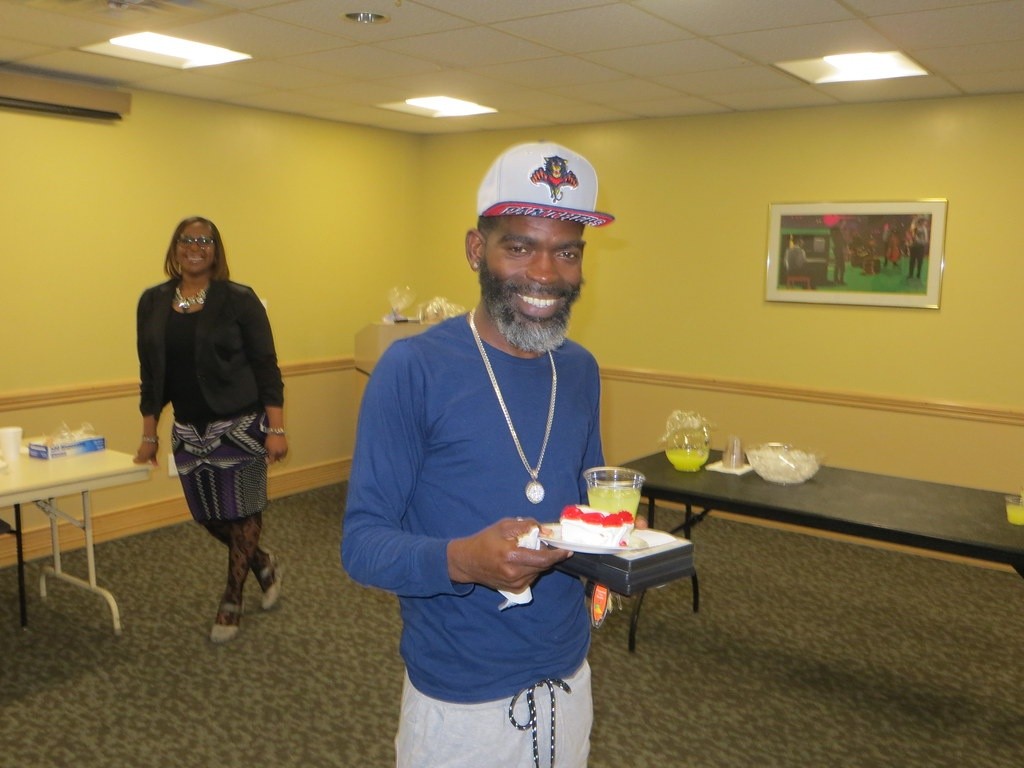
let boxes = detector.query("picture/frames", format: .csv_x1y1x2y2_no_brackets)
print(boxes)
765,199,950,314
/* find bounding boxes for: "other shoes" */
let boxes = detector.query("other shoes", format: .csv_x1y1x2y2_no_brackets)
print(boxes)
209,599,246,644
261,555,287,610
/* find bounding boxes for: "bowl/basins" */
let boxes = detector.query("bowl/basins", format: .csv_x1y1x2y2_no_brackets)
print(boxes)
746,440,828,484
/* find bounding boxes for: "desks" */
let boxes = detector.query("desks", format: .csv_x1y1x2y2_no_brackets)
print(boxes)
0,432,160,640
604,442,1024,656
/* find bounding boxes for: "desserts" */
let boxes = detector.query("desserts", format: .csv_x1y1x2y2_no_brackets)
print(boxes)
558,504,636,547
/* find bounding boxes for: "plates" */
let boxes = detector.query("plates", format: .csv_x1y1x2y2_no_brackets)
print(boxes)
536,520,676,554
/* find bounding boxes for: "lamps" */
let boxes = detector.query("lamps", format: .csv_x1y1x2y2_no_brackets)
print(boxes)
373,97,500,117
73,31,255,71
345,8,392,27
773,46,930,89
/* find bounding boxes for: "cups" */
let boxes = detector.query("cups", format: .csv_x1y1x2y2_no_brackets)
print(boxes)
0,426,24,462
721,433,745,468
666,426,709,471
583,466,646,521
1004,494,1024,526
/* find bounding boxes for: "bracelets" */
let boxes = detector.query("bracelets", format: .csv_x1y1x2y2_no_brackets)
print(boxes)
265,426,286,436
140,433,160,443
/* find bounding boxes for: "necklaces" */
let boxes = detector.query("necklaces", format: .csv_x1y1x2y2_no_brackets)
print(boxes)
469,305,557,505
173,286,206,308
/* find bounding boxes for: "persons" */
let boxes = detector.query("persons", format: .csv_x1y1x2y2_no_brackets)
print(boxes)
340,140,649,768
137,217,288,645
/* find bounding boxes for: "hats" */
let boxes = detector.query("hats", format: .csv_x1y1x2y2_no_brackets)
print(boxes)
476,142,615,230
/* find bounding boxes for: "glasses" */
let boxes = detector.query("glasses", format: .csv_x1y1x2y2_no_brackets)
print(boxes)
175,234,216,251
